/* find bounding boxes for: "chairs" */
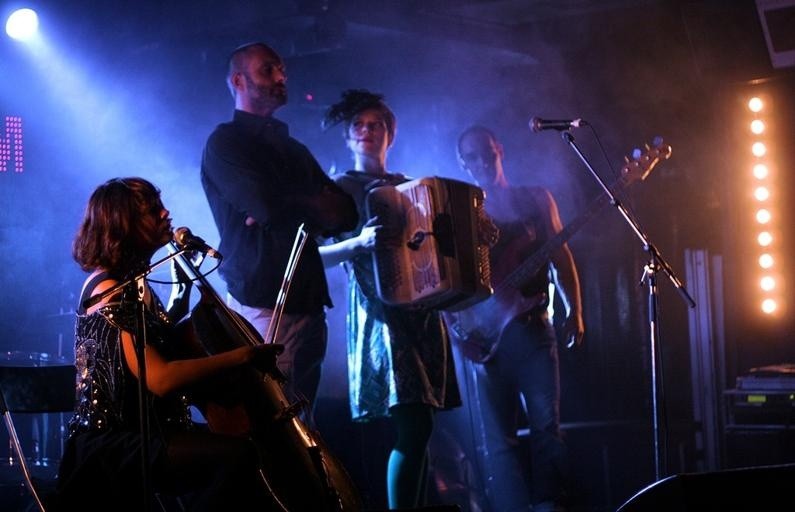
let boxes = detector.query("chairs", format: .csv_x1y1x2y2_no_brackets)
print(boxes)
0,366,187,512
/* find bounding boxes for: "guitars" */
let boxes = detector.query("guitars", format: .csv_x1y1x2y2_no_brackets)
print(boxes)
445,138,671,366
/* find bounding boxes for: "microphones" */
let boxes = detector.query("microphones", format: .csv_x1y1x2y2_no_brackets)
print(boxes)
528,116,582,132
174,227,221,260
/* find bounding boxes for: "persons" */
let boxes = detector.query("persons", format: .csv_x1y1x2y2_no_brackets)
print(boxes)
202,43,358,430
46,176,284,509
308,88,502,507
439,125,586,509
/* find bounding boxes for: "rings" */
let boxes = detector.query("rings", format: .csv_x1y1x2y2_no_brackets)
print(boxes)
490,236,495,242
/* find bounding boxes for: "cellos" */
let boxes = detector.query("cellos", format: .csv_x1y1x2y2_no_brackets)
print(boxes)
167,235,361,509
